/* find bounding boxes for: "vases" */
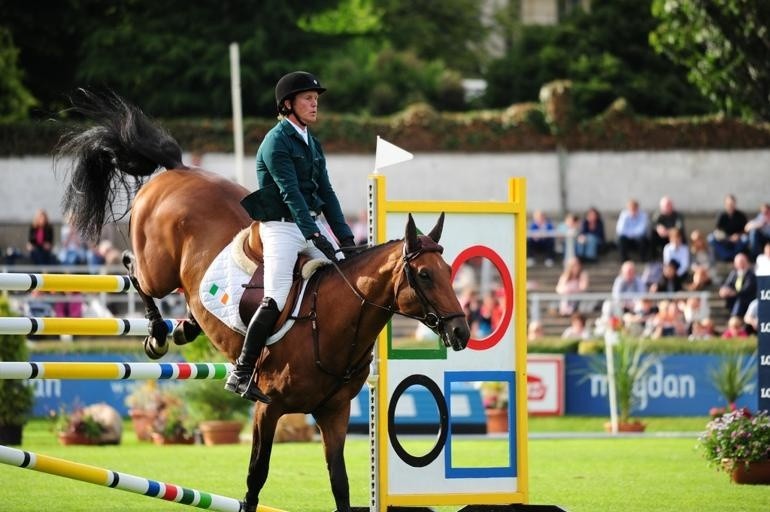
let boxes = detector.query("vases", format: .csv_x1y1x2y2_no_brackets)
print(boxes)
728,461,769,487
487,411,511,434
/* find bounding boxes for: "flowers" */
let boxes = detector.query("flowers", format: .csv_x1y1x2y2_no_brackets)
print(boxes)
480,380,509,411
700,403,769,475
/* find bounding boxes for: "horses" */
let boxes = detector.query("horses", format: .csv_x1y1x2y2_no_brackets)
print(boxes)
52,84,471,512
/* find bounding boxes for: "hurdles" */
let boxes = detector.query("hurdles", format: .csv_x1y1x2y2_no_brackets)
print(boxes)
0,272,290,512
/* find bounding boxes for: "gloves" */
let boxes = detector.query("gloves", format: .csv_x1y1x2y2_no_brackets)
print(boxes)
340,235,359,260
309,235,336,259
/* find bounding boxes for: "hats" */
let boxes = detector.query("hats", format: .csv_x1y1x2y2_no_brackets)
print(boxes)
273,69,329,117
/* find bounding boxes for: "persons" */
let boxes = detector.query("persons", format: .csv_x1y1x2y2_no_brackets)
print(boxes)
520,191,770,341
1,210,129,342
223,70,359,405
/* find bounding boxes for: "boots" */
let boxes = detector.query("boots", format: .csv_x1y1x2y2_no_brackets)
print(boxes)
224,295,286,405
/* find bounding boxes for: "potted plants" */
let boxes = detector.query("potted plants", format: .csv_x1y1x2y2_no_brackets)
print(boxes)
0,290,39,451
570,318,674,433
59,329,255,449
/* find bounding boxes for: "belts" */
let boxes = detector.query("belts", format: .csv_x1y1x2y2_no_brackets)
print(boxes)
256,206,322,228
